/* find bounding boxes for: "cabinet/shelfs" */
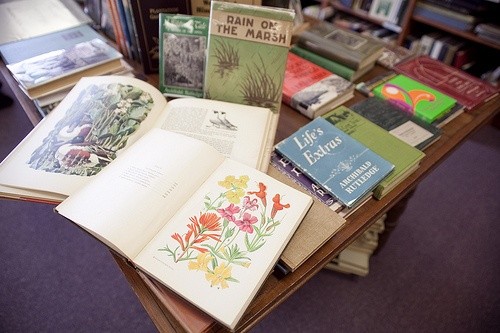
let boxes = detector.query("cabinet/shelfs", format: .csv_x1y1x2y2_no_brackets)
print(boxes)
321,0,500,88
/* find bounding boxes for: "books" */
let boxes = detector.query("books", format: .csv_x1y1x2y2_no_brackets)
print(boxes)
0,0,500,330
